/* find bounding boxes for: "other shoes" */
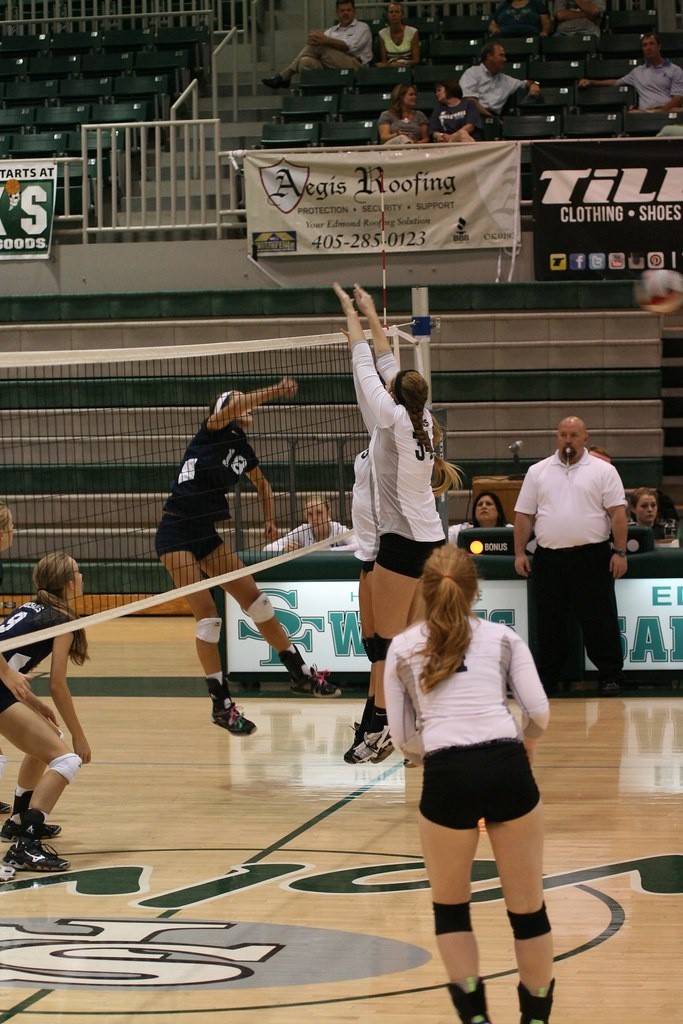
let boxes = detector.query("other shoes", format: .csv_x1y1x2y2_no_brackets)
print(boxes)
0,864,15,881
0,802,12,813
600,681,621,695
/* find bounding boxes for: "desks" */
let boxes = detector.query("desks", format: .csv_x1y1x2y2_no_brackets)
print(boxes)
212,547,683,696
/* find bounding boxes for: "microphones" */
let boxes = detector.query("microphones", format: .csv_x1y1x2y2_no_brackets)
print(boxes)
509,440,524,453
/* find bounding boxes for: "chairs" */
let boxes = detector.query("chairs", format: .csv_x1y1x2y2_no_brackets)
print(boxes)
261,0,683,191
0,22,213,215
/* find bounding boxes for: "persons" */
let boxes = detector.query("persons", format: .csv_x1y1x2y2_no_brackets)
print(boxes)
259,0,683,148
265,282,683,767
384,546,558,1024
0,504,93,888
155,373,344,738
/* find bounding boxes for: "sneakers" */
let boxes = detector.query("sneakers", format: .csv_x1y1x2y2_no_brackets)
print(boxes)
211,697,257,737
290,663,341,697
0,818,62,841
344,722,396,764
2,839,71,871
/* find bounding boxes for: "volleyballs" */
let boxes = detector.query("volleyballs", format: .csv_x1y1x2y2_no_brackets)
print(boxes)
635,269,683,313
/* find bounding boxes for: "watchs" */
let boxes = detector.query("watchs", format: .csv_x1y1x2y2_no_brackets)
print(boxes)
612,545,628,557
